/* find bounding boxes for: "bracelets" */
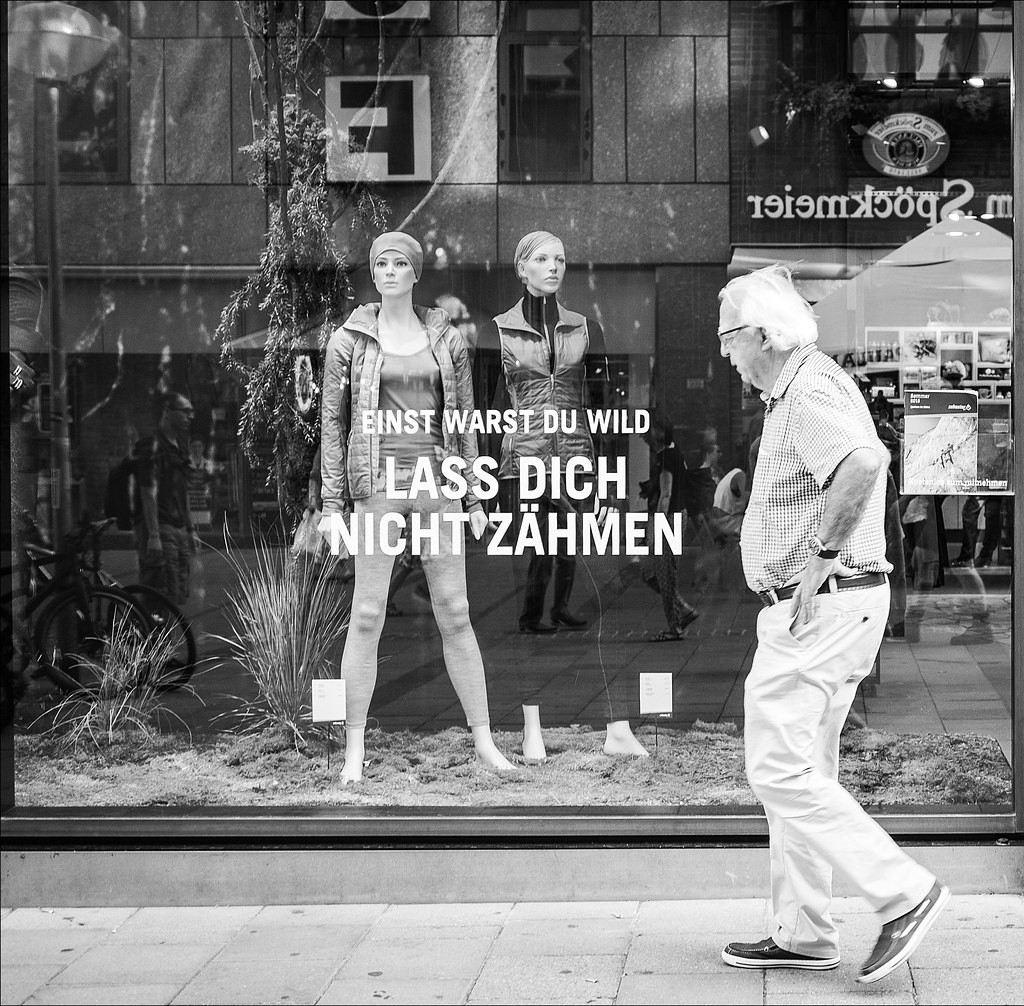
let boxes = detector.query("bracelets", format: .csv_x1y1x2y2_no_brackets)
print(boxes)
185,526,196,533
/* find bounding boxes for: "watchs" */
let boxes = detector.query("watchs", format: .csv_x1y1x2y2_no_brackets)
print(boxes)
807,534,841,559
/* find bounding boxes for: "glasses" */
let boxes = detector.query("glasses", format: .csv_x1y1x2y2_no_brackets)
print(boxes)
717,325,752,344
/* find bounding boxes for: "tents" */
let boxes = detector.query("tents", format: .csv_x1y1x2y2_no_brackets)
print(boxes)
812,214,1013,350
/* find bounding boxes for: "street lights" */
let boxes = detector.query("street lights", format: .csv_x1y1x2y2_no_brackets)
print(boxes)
4,1,118,700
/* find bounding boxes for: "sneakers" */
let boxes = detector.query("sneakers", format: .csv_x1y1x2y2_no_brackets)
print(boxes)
857,880,951,985
721,938,841,971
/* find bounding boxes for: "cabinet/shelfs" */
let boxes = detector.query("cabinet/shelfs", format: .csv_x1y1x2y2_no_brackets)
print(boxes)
864,326,1013,399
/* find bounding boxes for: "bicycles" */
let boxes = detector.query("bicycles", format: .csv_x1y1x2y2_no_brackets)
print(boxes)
12,508,197,698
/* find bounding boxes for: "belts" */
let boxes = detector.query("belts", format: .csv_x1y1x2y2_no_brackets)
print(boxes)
758,569,886,607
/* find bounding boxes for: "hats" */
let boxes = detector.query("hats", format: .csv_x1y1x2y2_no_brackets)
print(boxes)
369,232,424,284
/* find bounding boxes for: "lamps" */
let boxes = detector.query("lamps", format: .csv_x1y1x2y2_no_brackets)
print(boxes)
960,75,985,96
872,77,899,91
9,2,112,86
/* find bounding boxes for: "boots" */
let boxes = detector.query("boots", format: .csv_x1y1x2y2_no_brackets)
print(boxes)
884,611,923,642
950,614,993,646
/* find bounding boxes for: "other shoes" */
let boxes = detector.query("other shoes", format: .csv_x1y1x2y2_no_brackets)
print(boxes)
519,618,558,633
552,613,587,630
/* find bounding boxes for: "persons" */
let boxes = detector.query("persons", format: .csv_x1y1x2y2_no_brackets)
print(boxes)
883,385,1013,646
634,411,700,641
474,232,651,759
692,437,751,544
319,234,521,782
716,266,951,984
126,390,212,670
386,517,432,618
517,421,591,631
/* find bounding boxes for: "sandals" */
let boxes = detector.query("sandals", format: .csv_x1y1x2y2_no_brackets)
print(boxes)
648,631,685,642
679,608,700,630
